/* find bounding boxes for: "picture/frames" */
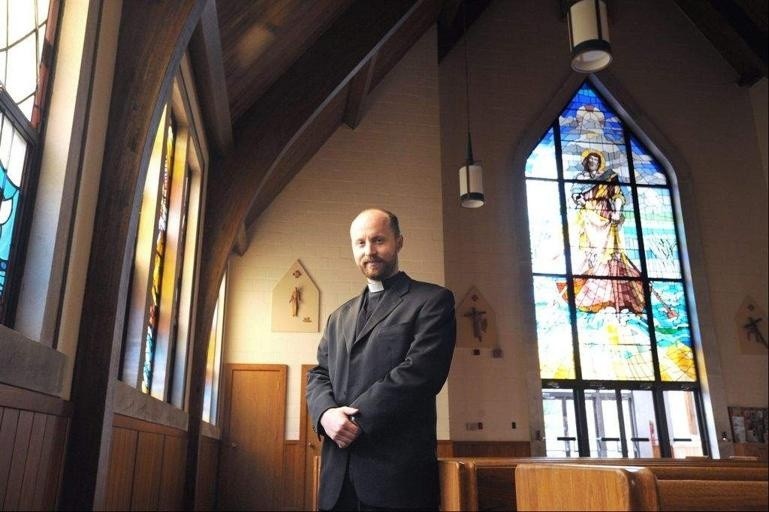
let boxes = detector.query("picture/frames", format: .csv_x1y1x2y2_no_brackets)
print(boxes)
728,406,769,443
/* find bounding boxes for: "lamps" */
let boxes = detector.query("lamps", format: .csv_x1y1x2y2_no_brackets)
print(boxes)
456,1,485,210
565,1,615,76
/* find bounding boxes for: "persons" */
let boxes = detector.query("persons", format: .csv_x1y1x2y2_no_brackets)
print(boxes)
556,103,696,377
304,208,456,511
